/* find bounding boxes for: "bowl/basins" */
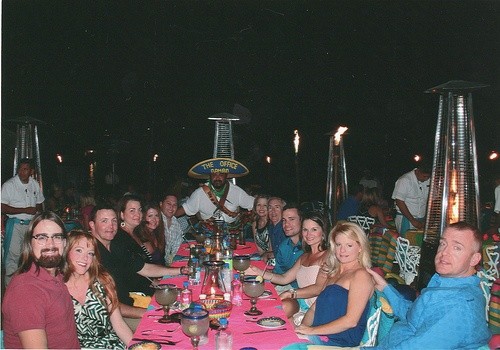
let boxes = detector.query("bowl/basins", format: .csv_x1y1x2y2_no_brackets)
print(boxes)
197,299,232,319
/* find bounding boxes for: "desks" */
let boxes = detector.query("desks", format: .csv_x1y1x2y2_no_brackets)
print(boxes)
127,240,305,350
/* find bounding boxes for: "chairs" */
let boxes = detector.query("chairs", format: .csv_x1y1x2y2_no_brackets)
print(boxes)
348,217,500,350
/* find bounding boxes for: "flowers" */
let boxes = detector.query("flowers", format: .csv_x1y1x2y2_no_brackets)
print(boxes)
238,212,251,232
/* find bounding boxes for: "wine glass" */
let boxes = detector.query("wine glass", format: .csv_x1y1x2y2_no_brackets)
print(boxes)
182,308,209,350
243,276,264,316
155,284,178,323
233,256,250,282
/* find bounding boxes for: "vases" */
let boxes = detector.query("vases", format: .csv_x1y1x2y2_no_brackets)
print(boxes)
240,230,246,245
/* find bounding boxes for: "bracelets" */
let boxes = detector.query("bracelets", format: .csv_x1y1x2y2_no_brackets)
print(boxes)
294,290,297,299
290,289,294,298
180,267,184,274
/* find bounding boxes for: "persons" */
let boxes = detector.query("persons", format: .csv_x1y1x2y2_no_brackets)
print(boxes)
336,186,387,236
294,221,374,347
237,213,332,328
2,211,81,350
270,202,305,295
56,229,135,350
1,158,45,290
111,195,192,297
360,221,493,350
392,160,432,237
46,184,96,231
87,205,148,332
136,204,166,265
158,192,184,264
175,171,256,243
250,193,287,264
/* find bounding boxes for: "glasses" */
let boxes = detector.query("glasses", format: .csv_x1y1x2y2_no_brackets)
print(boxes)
32,234,66,244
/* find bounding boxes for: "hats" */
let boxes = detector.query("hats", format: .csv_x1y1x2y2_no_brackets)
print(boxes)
187,158,250,178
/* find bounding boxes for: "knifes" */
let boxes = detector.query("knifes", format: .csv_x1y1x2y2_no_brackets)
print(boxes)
132,338,175,345
243,328,287,334
242,298,276,301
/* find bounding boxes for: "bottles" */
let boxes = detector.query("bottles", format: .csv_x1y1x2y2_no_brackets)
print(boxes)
230,274,243,306
192,305,207,344
180,282,191,309
216,318,233,350
189,258,200,286
201,220,246,299
188,246,201,280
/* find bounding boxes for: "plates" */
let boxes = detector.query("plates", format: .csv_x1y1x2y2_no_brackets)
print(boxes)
128,341,162,350
257,317,286,328
259,290,273,298
